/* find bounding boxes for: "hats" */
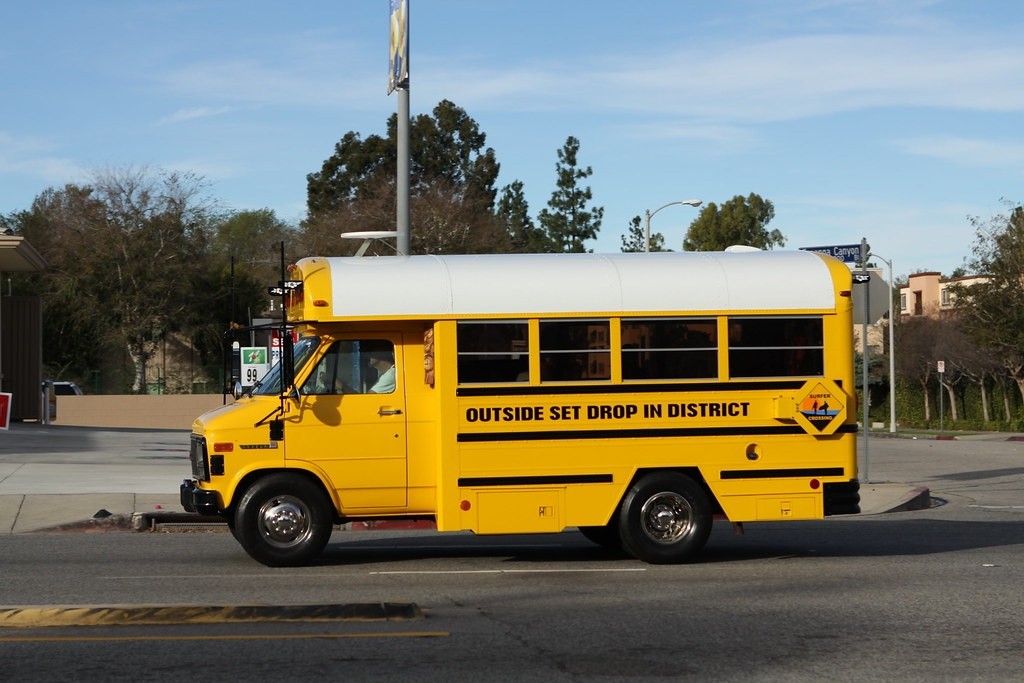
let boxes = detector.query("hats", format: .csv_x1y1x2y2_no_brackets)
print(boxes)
359,340,394,361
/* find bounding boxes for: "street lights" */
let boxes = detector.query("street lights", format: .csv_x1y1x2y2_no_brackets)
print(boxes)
647,197,703,254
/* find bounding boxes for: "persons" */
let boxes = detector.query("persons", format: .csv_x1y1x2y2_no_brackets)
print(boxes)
320,339,395,394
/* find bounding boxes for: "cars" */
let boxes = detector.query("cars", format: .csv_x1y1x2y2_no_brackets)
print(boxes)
41,379,83,396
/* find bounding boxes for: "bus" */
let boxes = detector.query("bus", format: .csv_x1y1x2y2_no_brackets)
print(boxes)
178,244,861,565
